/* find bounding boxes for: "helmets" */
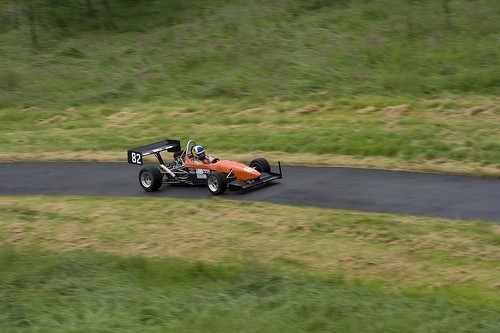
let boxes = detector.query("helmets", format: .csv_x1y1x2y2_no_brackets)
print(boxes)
192,146,205,160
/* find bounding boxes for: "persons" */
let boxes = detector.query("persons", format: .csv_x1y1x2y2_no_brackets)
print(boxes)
191,145,214,165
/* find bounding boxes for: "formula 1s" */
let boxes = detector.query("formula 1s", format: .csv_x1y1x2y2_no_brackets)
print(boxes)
127,139,283,195
